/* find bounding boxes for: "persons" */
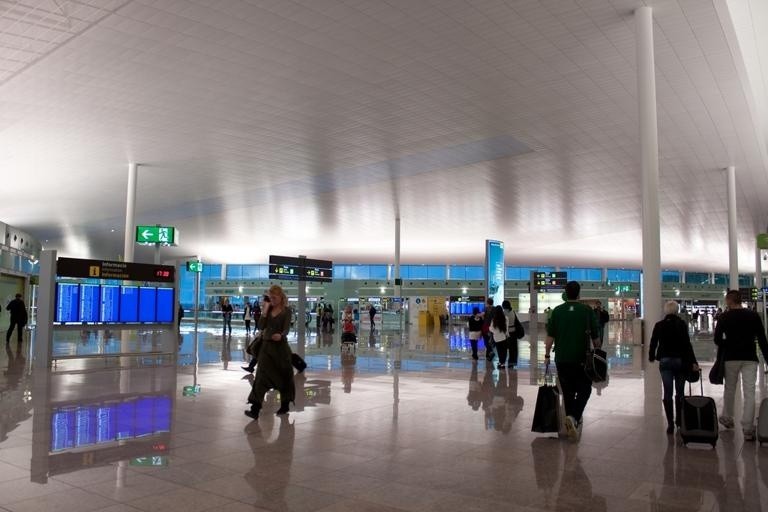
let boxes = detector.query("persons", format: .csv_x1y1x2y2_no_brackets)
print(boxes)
6,293,28,343
3,342,25,390
555,438,607,512
713,308,723,320
649,427,760,512
712,310,718,333
369,304,377,328
466,359,524,434
178,303,185,334
544,281,602,443
544,307,553,327
648,301,700,434
369,327,376,350
468,298,520,369
244,413,295,511
681,306,688,314
222,285,360,420
593,300,609,347
714,290,768,440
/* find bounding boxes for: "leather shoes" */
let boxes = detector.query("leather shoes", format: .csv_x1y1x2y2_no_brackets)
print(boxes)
245,411,258,420
276,404,290,415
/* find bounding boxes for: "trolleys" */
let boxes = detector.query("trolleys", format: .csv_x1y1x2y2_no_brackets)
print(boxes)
342,354,356,376
339,317,358,354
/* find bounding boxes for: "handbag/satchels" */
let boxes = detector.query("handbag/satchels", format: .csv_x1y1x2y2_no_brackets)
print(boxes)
682,358,700,383
531,385,560,433
513,317,525,340
584,348,608,383
708,357,724,385
246,334,263,358
531,436,561,489
343,322,353,332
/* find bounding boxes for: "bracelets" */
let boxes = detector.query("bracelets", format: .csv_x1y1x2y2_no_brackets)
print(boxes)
544,355,550,359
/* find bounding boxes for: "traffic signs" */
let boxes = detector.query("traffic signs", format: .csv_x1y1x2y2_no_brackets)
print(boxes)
534,273,568,287
270,256,332,282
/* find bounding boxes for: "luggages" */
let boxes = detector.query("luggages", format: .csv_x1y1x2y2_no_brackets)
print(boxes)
291,353,307,372
307,379,331,386
675,448,724,491
756,398,768,447
680,395,719,449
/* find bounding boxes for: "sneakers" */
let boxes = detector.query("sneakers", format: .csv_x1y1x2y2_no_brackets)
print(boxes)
719,415,735,429
563,415,579,444
744,431,753,441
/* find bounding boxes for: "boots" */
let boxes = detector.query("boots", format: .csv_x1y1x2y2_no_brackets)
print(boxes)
662,399,675,436
675,394,683,429
